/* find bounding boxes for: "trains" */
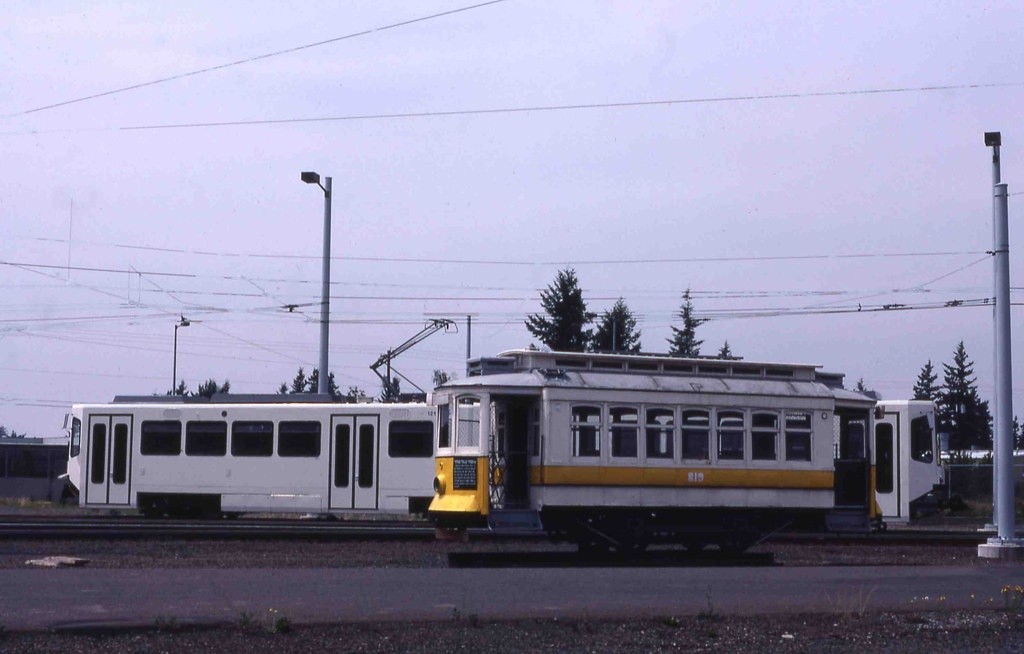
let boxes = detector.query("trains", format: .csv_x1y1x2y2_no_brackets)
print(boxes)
419,343,889,559
60,394,946,525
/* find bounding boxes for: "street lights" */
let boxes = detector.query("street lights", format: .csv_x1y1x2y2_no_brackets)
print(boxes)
171,321,190,395
981,129,1016,540
301,170,333,394
